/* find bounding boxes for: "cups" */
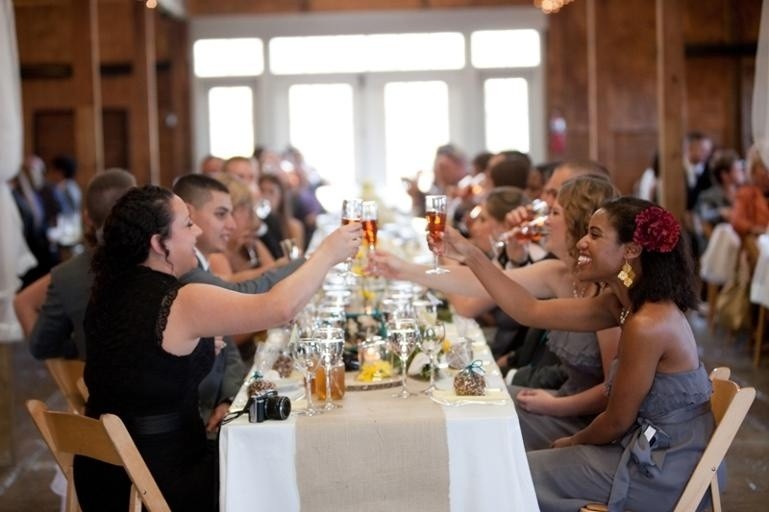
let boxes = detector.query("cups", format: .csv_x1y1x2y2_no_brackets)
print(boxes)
447,337,474,371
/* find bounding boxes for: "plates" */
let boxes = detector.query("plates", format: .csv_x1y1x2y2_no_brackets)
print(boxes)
344,370,406,390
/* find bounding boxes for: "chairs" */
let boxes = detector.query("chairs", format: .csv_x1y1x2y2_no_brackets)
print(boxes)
580,366,756,512
26,399,173,512
42,356,89,416
698,218,767,371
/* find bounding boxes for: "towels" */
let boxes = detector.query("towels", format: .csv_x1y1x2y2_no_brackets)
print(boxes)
429,385,507,403
261,369,305,403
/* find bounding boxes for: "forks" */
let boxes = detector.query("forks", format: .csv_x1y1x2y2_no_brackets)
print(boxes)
430,396,508,408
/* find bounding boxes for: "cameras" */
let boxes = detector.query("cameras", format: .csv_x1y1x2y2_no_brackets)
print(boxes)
248,389,291,423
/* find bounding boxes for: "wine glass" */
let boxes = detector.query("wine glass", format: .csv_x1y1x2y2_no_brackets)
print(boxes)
292,338,324,417
424,194,450,275
531,199,548,219
385,318,417,399
417,320,446,396
313,327,346,411
381,277,438,320
341,198,362,225
487,216,549,257
315,262,352,327
362,202,383,273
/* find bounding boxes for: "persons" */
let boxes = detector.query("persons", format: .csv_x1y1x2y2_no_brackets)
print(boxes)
424,195,728,512
73,184,366,511
400,130,769,347
359,175,622,450
12,147,331,441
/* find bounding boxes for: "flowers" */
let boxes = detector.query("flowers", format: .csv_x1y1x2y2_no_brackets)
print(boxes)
633,205,681,255
